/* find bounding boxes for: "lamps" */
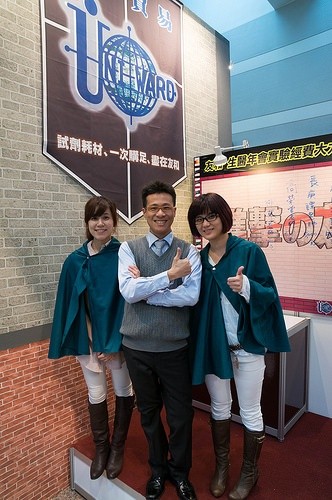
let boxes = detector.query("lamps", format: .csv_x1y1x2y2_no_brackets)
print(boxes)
212,140,248,167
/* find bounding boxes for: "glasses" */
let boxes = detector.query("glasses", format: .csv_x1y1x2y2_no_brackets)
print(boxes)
146,207,175,212
194,212,218,225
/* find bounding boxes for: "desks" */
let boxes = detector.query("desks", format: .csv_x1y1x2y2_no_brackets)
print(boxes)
192,313,311,442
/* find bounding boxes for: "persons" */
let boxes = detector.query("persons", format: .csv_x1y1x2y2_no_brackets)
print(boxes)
47,196,135,480
187,193,291,500
118,179,202,500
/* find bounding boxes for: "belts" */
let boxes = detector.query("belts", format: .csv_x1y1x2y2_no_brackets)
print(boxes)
228,343,242,369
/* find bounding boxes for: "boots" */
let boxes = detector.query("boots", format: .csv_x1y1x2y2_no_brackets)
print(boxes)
106,394,135,480
88,398,111,480
229,425,265,500
209,414,232,497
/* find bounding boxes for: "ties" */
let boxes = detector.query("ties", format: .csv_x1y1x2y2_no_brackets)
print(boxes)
153,241,167,257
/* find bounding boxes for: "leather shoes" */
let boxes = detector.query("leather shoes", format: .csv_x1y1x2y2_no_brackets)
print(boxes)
145,475,166,500
170,479,197,500
